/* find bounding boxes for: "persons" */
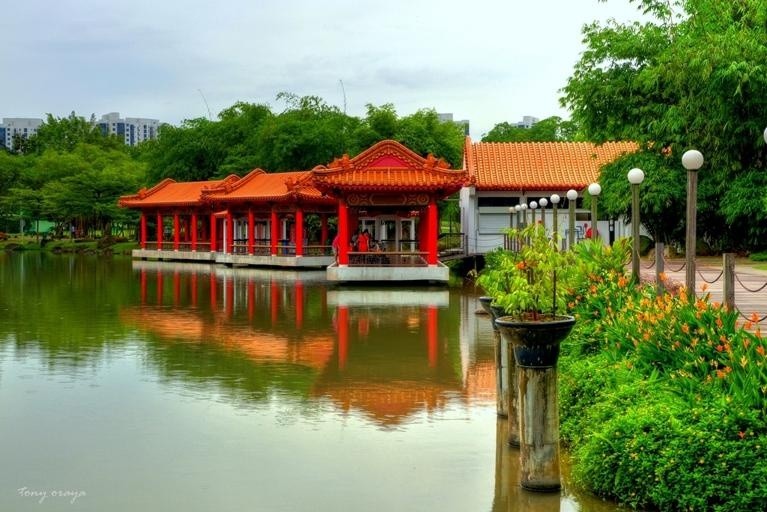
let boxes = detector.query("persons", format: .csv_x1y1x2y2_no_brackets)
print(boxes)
332,228,384,267
582,223,590,239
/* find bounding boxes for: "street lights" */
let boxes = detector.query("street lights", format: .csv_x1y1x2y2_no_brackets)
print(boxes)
564,187,579,252
549,194,562,252
627,168,645,285
588,183,602,250
679,149,705,305
506,199,550,254
